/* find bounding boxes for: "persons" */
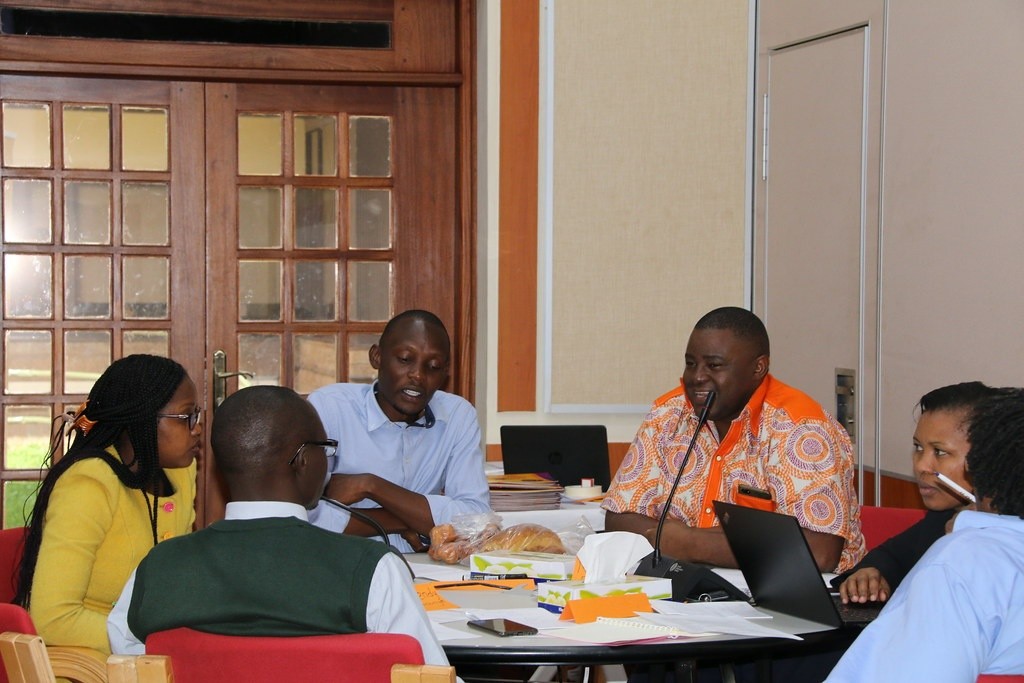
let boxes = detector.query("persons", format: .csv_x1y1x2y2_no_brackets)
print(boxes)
12,354,202,656
107,385,465,683
304,309,491,555
829,381,994,603
824,386,1024,683
598,306,870,682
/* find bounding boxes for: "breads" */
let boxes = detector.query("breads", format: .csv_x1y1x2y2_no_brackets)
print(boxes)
428,521,564,565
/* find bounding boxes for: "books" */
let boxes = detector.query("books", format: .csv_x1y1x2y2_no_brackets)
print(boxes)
486,473,564,512
547,616,717,648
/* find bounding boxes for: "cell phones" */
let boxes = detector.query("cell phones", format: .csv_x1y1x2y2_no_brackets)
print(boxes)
467,618,538,638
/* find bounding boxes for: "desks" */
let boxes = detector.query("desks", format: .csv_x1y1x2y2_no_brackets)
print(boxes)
495,493,606,533
413,567,856,683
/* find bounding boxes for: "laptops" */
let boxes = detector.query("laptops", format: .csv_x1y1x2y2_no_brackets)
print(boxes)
712,500,891,628
500,425,611,495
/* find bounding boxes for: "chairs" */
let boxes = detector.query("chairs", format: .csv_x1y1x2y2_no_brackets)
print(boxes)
0,525,109,683
105,628,457,683
859,505,928,551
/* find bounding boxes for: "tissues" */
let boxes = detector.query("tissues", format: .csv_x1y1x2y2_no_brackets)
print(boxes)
537,529,673,614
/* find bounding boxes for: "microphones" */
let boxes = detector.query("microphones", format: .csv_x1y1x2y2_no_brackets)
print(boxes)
633,390,756,603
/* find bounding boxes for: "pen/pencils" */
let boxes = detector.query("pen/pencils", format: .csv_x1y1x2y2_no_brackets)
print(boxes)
935,472,976,503
472,574,528,580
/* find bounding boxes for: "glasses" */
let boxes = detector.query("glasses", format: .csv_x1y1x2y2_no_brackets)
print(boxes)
288,438,339,466
156,407,202,430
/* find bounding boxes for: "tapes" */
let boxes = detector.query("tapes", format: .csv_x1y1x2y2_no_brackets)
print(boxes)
565,485,602,499
